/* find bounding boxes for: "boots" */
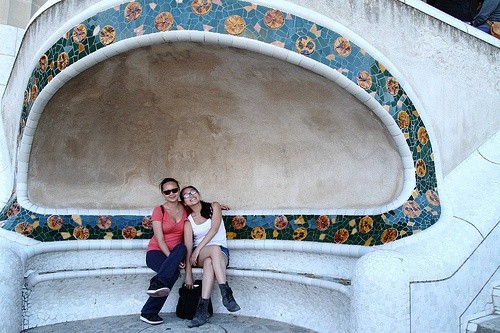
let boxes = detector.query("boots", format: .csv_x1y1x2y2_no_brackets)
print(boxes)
186,297,210,328
219,282,240,312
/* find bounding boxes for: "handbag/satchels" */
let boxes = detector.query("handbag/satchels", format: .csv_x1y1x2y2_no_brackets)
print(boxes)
175,280,213,319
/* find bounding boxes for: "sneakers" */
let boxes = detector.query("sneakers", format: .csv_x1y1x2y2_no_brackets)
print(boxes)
147,280,171,297
140,312,163,324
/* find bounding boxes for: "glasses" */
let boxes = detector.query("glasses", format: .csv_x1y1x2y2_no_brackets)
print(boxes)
163,188,179,195
182,189,197,198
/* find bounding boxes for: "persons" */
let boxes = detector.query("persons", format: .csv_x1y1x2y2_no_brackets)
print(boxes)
140,177,230,324
180,185,241,327
427,0,472,24
465,0,500,40
